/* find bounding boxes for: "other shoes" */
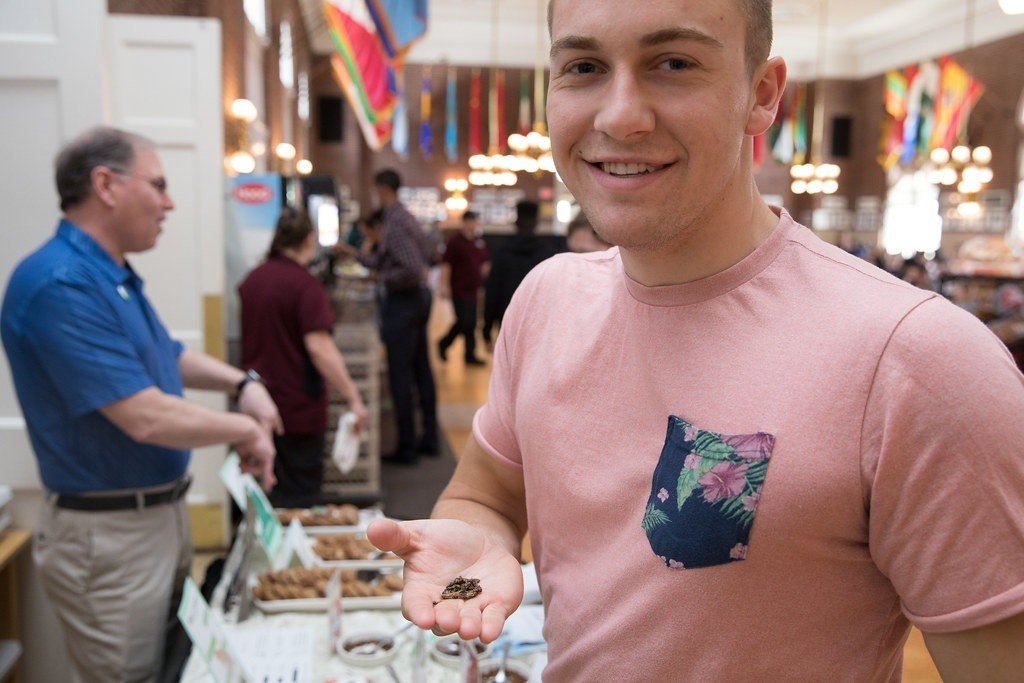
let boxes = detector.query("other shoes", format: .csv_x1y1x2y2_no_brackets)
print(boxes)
438,340,447,361
384,447,415,466
416,437,439,456
465,355,485,365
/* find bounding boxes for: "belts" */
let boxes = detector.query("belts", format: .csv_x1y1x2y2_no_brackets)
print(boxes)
53,474,192,510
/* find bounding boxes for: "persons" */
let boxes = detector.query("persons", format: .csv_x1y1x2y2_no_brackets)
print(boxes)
367,1,1024,683
439,199,615,366
0,127,284,683
236,208,367,509
334,169,440,468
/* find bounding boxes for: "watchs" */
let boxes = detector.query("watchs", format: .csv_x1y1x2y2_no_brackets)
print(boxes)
237,368,262,398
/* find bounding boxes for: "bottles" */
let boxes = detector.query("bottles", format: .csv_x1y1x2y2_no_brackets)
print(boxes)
316,631,407,683
426,636,493,683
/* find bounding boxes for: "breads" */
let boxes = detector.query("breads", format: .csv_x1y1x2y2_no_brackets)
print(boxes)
312,535,402,560
250,566,404,600
277,503,358,524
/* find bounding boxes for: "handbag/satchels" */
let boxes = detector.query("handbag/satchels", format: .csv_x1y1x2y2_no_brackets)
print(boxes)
333,415,361,474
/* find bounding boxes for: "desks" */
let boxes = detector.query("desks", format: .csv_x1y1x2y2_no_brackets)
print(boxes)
157,507,547,683
